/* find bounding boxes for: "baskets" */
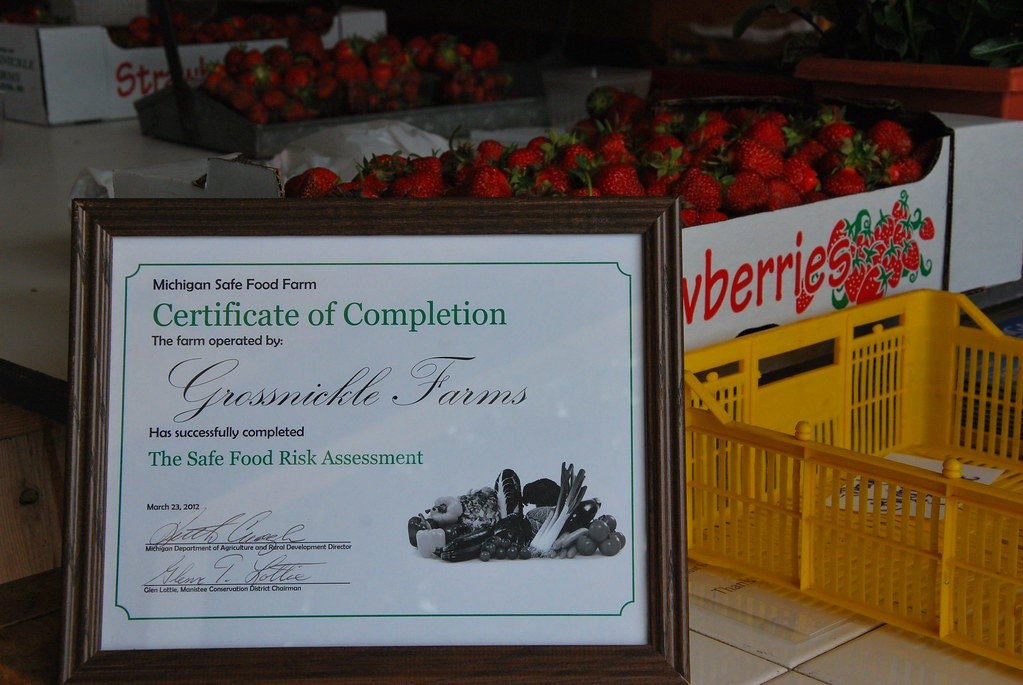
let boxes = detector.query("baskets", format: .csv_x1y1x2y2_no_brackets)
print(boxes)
685,288,1023,685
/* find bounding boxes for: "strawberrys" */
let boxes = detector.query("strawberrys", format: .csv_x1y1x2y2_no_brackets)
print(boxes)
0,0,522,125
285,81,924,231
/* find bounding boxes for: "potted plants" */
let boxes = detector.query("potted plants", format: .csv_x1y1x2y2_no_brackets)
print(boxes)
728,0,1023,124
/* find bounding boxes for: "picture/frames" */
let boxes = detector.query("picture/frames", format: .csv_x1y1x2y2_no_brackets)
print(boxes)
60,197,692,685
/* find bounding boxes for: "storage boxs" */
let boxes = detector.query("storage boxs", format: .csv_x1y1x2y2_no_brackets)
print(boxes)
106,96,1023,671
1,1,388,128
